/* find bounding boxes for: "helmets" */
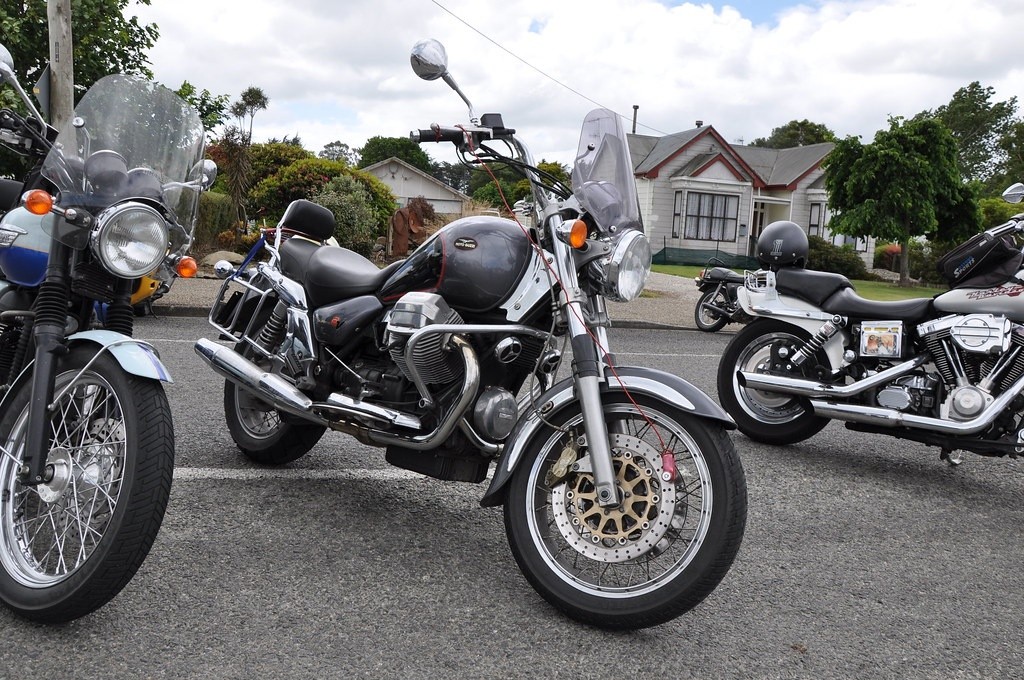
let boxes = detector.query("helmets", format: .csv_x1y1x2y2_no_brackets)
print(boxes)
757,221,808,272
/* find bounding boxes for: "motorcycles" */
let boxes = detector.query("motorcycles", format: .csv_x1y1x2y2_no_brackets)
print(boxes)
694,257,754,331
714,181,1024,465
0,47,219,621
191,41,751,632
